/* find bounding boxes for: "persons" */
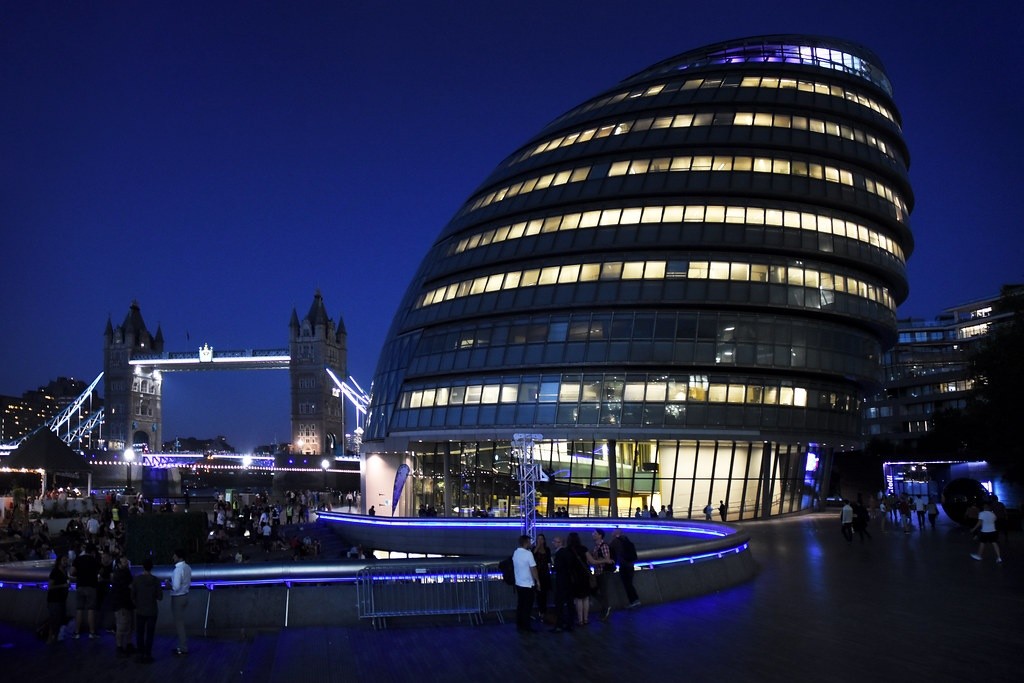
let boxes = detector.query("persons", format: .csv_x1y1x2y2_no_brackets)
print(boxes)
547,535,574,632
591,528,616,621
165,549,192,657
610,527,643,609
838,481,1010,544
969,501,1004,564
203,487,673,577
0,483,190,640
563,532,607,626
512,535,541,633
530,534,553,624
132,559,163,664
705,500,728,522
42,553,74,645
111,555,137,658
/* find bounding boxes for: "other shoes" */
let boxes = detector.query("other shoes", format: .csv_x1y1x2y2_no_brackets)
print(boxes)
970,554,982,560
125,643,137,652
72,632,80,638
566,626,574,631
116,651,129,658
172,649,180,652
174,651,188,656
996,559,1002,563
549,627,562,632
88,632,100,638
625,603,632,608
630,602,642,607
601,607,613,620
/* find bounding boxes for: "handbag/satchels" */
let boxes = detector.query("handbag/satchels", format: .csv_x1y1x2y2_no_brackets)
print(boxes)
590,572,596,587
543,610,561,624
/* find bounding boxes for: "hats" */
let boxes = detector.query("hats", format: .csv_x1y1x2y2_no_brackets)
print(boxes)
843,498,848,500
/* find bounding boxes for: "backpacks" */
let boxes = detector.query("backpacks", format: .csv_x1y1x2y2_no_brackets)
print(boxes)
598,542,617,572
704,506,708,513
498,558,515,586
